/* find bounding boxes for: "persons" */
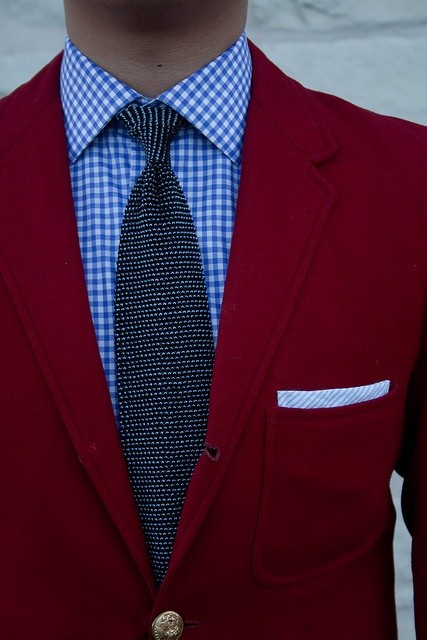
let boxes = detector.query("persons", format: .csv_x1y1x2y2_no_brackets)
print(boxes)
0,1,427,640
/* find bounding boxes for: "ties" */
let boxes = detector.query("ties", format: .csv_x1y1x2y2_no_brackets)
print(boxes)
114,104,215,588
